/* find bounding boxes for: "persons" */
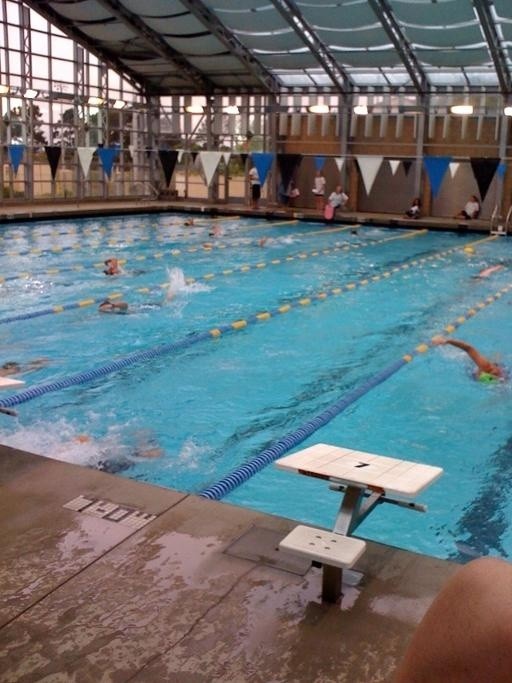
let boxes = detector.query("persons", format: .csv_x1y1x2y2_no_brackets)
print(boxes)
0,358,51,378
453,195,480,220
207,223,223,238
323,185,350,226
97,269,179,313
311,168,326,212
184,215,194,225
287,178,302,207
248,165,262,209
101,256,122,275
431,335,511,383
378,554,511,683
401,197,422,220
